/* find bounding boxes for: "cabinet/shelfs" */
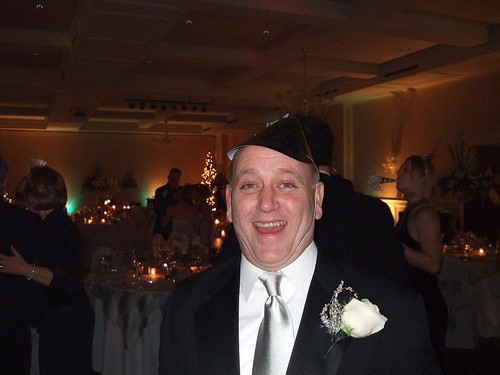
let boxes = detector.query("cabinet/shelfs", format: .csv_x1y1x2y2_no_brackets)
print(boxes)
380,198,464,244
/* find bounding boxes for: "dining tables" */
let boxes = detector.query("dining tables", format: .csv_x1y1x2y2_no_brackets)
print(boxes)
437,252,499,348
76,222,147,269
82,262,212,375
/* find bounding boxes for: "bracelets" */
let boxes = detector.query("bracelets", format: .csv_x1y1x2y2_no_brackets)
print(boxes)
27,263,36,280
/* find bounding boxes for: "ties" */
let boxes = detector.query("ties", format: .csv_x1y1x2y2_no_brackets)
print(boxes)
252,272,295,375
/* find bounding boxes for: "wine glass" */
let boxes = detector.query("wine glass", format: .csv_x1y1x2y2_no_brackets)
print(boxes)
195,254,203,274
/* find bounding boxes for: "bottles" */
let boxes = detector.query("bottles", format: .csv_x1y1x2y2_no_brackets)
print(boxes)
106,250,111,273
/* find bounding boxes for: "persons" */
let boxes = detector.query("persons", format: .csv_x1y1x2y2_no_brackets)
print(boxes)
0,154,46,375
153,168,183,240
392,152,448,374
161,184,200,247
0,165,95,375
290,116,438,375
160,111,445,375
188,184,216,242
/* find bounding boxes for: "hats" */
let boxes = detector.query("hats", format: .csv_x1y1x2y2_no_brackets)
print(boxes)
226,116,316,166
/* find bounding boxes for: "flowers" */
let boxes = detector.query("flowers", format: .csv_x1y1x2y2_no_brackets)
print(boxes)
317,281,389,358
81,160,109,195
